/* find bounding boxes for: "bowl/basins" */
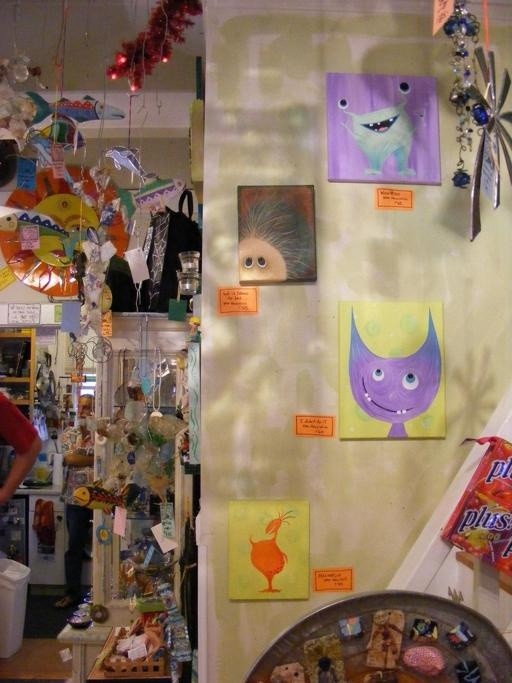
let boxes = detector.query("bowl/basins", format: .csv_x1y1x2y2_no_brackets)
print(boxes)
66,593,94,629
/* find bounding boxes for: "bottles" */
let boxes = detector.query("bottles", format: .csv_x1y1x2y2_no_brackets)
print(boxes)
160,502,174,537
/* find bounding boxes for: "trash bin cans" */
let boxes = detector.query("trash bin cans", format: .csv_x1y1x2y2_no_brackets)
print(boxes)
0,557,31,659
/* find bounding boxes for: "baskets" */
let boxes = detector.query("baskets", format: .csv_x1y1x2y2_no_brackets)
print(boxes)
102,624,166,678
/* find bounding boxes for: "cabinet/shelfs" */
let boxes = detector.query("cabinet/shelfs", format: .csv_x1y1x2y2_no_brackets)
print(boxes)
58,341,191,679
1,327,35,421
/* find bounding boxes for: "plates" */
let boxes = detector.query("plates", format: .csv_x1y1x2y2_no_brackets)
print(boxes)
23,479,52,488
0,166,132,297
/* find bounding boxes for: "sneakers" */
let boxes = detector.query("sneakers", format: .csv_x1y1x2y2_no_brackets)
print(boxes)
55,594,80,609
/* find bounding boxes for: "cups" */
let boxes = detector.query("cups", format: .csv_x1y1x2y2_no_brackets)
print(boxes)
176,252,200,294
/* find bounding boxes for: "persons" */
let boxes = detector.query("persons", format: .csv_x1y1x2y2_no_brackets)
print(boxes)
0,389,42,503
50,393,94,607
